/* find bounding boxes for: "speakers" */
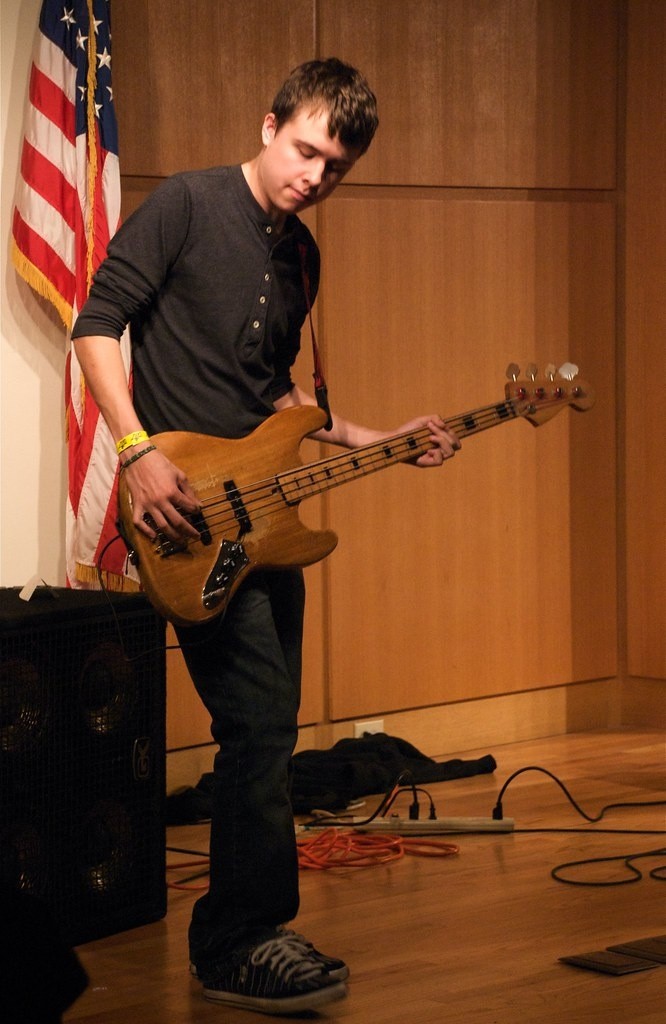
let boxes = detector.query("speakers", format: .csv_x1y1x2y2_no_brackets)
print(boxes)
0,583,167,945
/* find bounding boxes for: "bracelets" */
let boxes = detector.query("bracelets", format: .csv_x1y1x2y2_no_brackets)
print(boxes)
116,431,156,468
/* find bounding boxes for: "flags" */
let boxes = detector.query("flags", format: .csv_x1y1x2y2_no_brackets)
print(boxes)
10,0,138,593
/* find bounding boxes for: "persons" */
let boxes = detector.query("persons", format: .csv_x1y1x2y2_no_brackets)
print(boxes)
71,57,461,1011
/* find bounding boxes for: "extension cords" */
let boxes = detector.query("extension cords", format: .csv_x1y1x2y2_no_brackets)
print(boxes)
353,816,514,837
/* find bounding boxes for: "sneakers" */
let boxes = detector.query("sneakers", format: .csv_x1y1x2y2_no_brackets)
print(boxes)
190,925,350,983
200,923,349,1013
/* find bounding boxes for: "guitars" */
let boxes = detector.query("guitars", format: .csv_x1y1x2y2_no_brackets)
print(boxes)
119,360,596,626
